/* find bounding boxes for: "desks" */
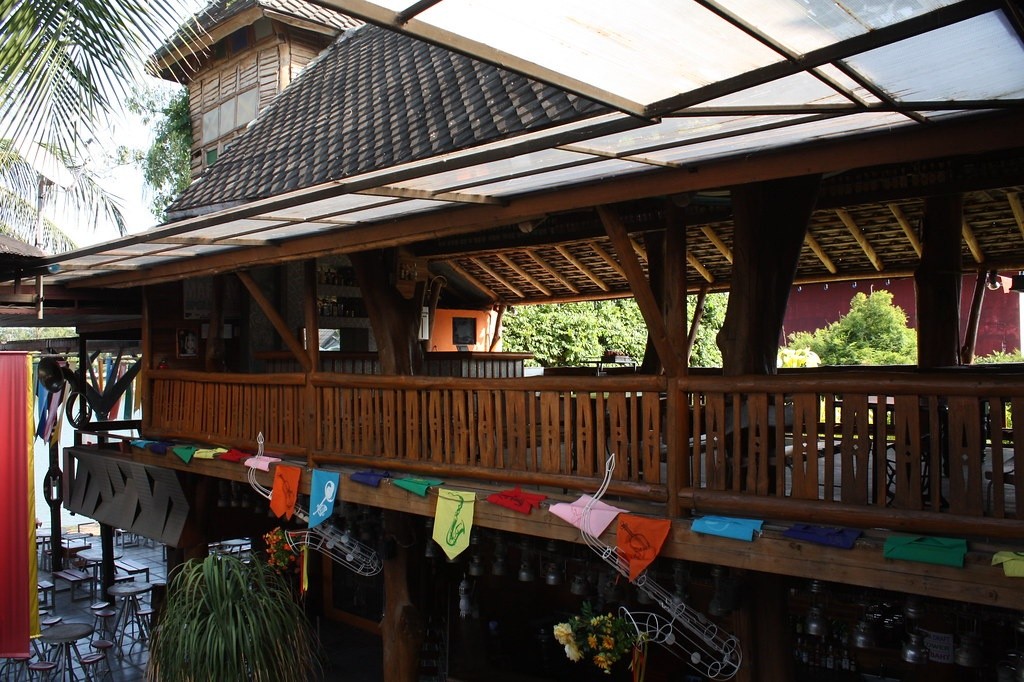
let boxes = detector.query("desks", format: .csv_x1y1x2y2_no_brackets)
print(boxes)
221,540,251,558
34,526,67,571
77,548,124,597
833,400,952,510
107,581,154,658
60,532,93,574
37,623,95,682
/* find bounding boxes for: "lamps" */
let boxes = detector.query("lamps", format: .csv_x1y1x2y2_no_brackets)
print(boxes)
456,569,471,619
446,554,465,564
469,526,487,577
545,539,563,586
706,567,732,617
672,560,693,599
492,529,513,577
424,518,440,558
636,558,658,604
986,269,1001,291
600,565,623,602
568,545,592,596
517,535,539,581
217,480,309,525
326,504,386,542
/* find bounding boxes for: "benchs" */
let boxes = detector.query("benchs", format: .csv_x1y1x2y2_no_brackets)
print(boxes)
96,574,135,600
212,547,251,564
72,561,102,586
52,569,94,602
114,556,150,594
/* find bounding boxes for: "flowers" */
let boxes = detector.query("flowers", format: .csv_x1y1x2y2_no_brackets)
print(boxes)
551,601,637,674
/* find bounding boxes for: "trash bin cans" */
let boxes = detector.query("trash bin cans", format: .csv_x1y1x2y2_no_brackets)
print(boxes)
150,582,167,630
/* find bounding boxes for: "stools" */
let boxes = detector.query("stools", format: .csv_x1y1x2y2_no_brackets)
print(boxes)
115,529,139,549
162,544,167,562
120,595,143,633
0,640,114,682
96,609,126,661
127,608,157,655
37,580,56,612
39,610,49,617
42,615,63,627
36,538,51,572
91,601,111,639
142,536,162,549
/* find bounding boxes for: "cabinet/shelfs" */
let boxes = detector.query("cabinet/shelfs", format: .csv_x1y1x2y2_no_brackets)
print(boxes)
277,261,378,353
